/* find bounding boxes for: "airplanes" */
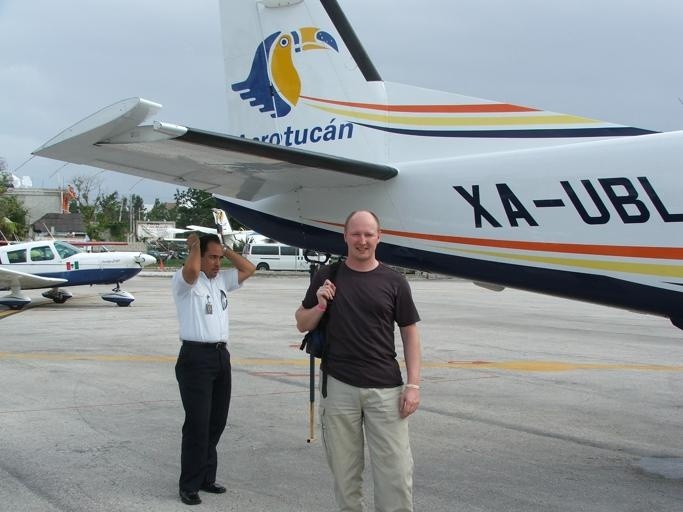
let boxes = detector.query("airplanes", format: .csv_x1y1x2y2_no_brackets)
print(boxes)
183,208,271,250
31,0,683,337
1,241,156,308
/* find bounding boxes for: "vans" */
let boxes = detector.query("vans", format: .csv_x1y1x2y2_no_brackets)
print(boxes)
242,244,330,275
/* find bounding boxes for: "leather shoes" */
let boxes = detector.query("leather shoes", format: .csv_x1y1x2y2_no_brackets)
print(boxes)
179,490,202,505
203,483,225,494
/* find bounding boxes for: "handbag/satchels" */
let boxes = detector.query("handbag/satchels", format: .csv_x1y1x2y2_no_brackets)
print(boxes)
303,260,339,358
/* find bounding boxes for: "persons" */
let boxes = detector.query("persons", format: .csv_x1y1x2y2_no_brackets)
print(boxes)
170,229,257,506
293,207,423,512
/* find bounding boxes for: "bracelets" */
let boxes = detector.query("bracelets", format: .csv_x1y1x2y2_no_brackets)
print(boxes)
404,382,421,391
221,246,227,256
315,303,326,313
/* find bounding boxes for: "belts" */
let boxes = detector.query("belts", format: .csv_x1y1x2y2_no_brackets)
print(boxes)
184,340,227,349
